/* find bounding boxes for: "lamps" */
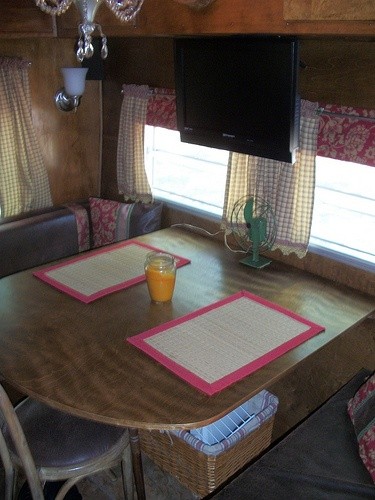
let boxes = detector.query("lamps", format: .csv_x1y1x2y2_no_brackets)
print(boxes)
35,0,145,62
54,67,89,113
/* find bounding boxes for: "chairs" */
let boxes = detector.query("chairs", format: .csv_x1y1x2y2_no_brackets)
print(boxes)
0,387,149,500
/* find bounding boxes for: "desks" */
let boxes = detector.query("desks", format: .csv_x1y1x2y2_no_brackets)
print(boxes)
0,226,375,432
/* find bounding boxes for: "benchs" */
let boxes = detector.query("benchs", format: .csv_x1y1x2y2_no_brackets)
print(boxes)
0,202,101,279
207,368,375,500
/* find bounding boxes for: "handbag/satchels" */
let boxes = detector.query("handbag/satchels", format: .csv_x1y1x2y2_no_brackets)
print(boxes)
347,374,375,483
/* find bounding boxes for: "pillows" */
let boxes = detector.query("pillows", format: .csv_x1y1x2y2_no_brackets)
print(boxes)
347,375,375,484
89,198,167,249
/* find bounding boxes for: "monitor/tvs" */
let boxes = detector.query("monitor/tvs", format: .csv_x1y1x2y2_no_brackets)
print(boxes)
172,31,301,167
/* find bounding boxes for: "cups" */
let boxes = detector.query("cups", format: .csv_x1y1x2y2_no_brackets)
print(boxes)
144,251,176,304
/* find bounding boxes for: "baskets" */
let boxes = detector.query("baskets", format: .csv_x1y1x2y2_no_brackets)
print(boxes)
138,389,278,499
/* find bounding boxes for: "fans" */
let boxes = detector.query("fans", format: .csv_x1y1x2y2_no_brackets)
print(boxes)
224,195,280,269
